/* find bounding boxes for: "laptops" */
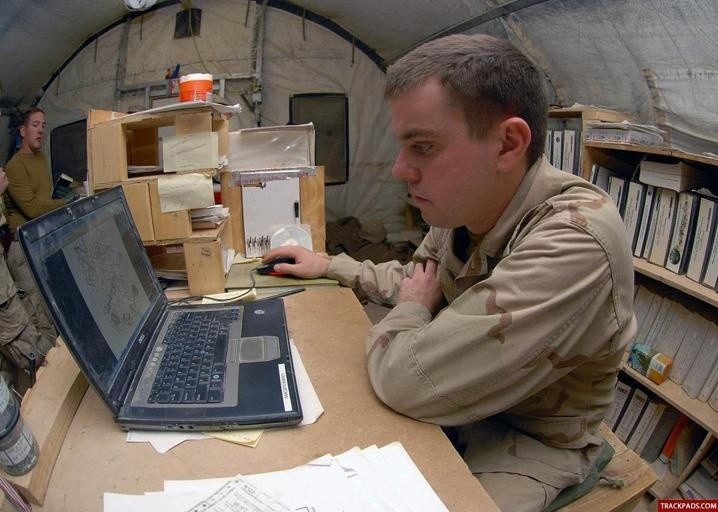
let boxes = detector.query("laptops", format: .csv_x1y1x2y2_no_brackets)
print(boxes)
16,185,303,432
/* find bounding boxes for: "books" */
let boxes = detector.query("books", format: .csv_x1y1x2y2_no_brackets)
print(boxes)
125,155,235,282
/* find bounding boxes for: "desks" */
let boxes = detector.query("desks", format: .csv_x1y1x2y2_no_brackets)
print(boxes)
0,254,501,512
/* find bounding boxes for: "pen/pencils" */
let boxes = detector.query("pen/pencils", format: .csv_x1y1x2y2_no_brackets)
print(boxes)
258,288,306,300
295,202,299,224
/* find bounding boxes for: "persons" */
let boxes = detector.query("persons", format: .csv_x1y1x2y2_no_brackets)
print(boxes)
0,107,89,386
544,104,717,511
261,33,640,512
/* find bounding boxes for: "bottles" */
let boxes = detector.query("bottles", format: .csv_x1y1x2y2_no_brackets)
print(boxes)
180,72,213,104
0,373,40,477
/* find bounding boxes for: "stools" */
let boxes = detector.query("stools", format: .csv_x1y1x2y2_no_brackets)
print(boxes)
550,419,658,512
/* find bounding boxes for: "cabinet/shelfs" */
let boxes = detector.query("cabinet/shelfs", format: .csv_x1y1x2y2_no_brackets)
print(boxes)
545,107,718,512
